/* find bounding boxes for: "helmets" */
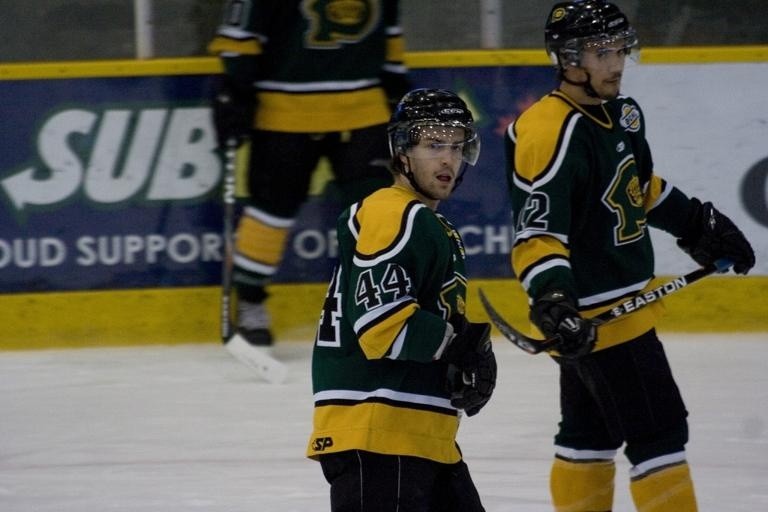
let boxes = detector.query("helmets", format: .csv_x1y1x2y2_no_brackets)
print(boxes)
543,1,639,69
388,87,481,166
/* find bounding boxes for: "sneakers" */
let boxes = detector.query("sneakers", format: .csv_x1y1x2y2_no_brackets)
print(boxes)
234,285,272,345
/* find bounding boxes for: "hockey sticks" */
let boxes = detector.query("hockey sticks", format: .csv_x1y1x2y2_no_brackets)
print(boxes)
477,256,734,355
216,96,287,385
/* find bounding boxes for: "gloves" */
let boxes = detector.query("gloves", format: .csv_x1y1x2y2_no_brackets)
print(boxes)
445,316,497,417
210,73,258,149
676,196,754,274
527,280,598,361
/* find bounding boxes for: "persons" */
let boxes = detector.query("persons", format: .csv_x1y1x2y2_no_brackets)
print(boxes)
503,1,755,512
305,87,496,511
208,1,407,347
